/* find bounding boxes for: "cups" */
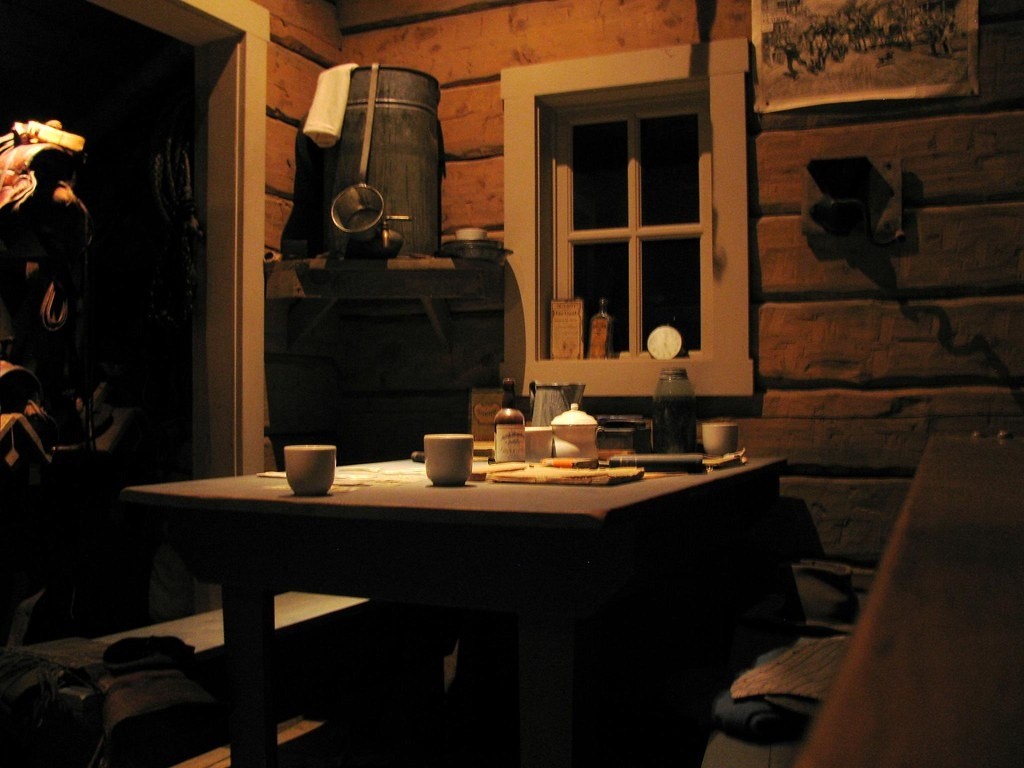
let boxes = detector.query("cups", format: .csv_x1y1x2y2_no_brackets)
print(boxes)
457,228,487,240
424,433,474,486
525,427,553,462
285,445,336,495
551,403,605,459
702,423,738,454
653,368,696,454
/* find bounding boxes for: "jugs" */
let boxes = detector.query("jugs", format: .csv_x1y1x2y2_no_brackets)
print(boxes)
529,380,586,426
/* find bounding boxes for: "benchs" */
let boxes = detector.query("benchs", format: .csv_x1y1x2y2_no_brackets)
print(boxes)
0,591,371,768
701,637,851,767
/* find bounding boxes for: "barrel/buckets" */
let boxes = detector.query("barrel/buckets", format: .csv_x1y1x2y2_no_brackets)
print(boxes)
321,64,440,254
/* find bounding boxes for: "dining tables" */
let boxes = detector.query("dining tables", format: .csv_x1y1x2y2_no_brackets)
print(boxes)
122,458,791,768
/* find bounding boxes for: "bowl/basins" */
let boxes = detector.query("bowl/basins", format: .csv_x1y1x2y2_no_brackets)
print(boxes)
441,241,502,256
452,247,513,266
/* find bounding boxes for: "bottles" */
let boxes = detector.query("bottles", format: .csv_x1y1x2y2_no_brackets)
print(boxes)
587,300,614,359
494,378,526,463
